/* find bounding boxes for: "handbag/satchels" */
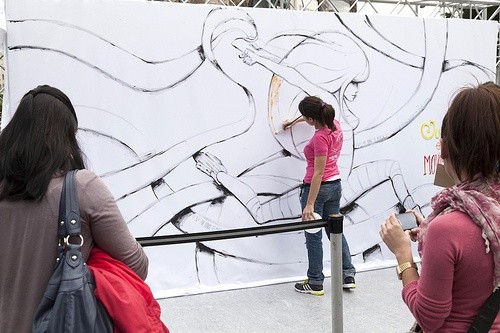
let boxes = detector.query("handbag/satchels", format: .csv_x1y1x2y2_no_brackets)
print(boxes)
31,168,114,332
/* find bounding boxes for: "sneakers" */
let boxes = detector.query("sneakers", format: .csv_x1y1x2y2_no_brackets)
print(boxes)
294,279,324,295
343,275,357,288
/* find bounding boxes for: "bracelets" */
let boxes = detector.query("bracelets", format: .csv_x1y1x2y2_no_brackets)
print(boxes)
396,261,418,280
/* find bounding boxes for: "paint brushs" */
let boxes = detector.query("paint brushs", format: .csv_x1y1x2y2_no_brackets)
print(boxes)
275,114,303,135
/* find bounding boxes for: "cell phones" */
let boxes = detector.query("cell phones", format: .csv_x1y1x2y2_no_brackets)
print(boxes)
395,212,418,231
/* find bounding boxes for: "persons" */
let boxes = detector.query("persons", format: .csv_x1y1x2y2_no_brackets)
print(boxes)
379,80,500,333
282,96,357,295
0,85,149,333
